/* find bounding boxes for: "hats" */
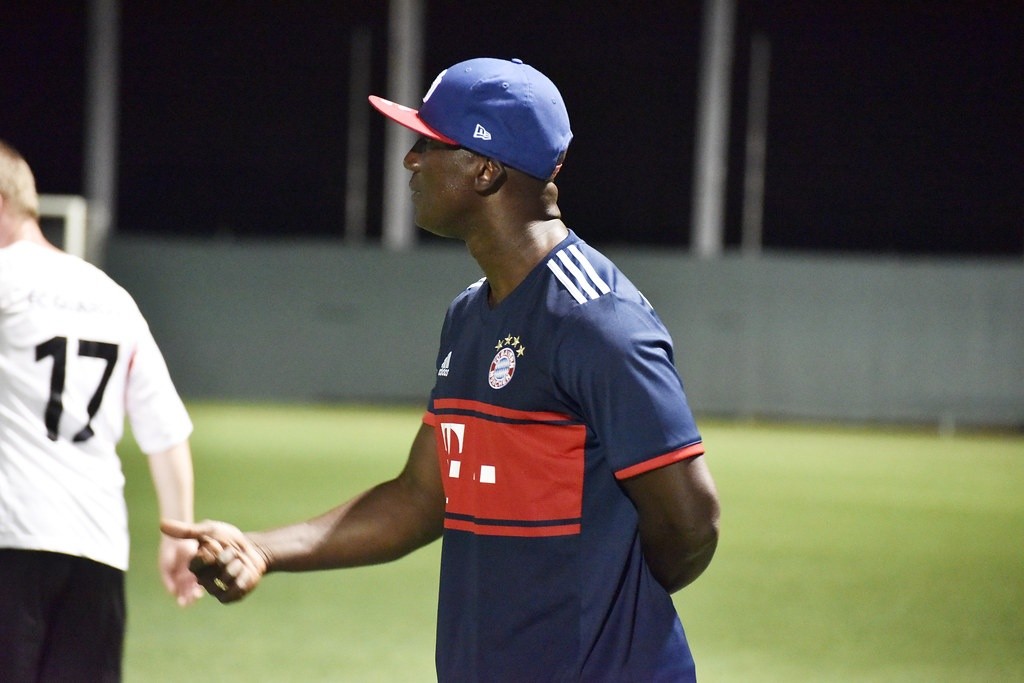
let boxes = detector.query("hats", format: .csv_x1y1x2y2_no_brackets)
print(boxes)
368,55,574,182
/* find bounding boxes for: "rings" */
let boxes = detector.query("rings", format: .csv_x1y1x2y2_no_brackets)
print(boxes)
214,578,228,591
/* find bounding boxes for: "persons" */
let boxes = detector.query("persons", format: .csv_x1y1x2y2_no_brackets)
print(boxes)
158,57,720,683
0,143,204,683
